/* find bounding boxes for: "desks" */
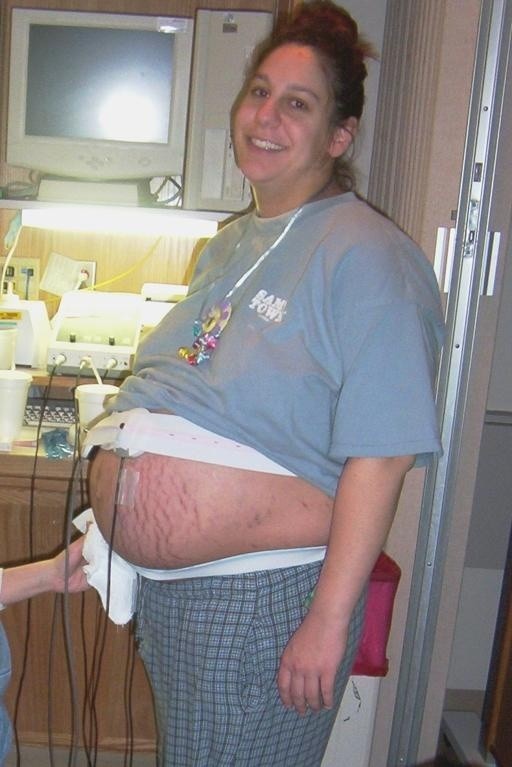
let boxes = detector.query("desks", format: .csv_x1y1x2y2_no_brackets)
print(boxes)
2,401,160,754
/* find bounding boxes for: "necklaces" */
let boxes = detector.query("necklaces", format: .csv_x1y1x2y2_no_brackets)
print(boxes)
190,175,335,338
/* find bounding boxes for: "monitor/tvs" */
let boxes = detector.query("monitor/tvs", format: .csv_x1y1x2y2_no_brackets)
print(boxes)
5,7,196,205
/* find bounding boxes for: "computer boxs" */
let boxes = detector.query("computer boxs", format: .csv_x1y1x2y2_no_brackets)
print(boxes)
183,8,276,215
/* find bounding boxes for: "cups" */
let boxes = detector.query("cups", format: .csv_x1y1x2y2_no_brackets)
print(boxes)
74,383,120,433
0,369,33,444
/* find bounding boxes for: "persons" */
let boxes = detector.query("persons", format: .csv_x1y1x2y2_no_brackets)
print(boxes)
86,0,454,765
1,517,94,612
